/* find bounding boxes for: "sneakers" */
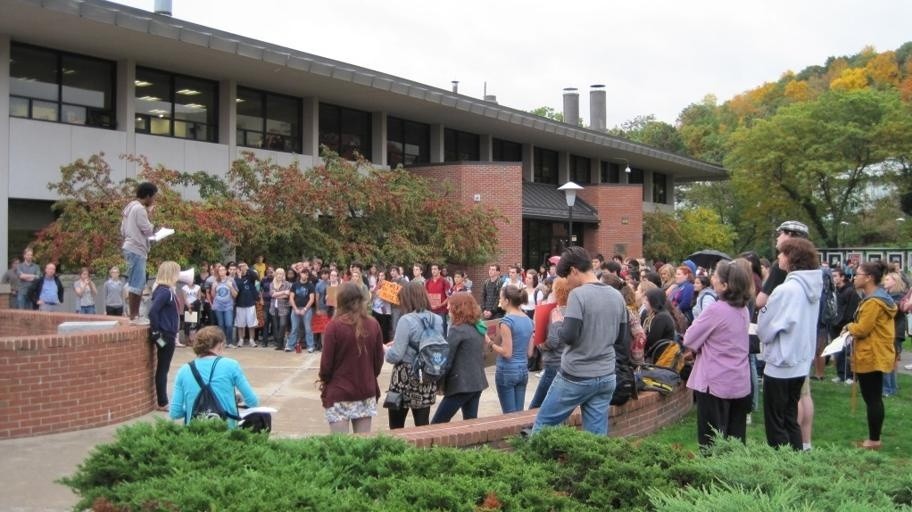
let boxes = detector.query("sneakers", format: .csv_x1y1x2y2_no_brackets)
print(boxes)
248,338,257,347
852,440,881,449
845,379,854,385
127,314,151,325
832,377,841,383
237,339,244,347
228,344,237,349
285,347,291,350
308,347,314,353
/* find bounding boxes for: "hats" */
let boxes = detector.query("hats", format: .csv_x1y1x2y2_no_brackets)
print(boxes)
776,221,809,235
683,259,696,277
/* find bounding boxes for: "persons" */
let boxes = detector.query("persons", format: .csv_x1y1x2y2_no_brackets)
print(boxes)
759,257,771,286
249,269,265,345
613,256,625,271
840,261,897,448
227,262,236,282
547,266,559,286
320,285,385,435
285,258,337,280
756,221,815,451
692,276,718,320
205,263,223,328
735,250,760,411
591,254,604,279
330,272,339,288
269,268,291,350
398,266,410,283
461,272,472,294
169,327,273,440
684,260,751,458
311,271,332,351
17,248,42,311
284,269,314,353
169,291,185,347
260,267,281,347
659,264,678,297
614,255,661,279
208,264,214,276
479,264,507,320
366,264,377,290
430,294,488,424
410,264,426,289
1,258,21,309
810,265,835,380
182,279,202,347
372,271,390,344
528,246,627,445
441,267,454,290
424,264,447,341
484,285,534,415
232,263,258,347
634,280,658,329
352,272,372,323
642,289,674,361
892,260,910,342
28,263,64,313
518,267,527,286
383,282,448,430
73,267,97,315
843,260,852,282
601,276,644,362
500,267,525,314
352,263,370,291
669,265,692,325
212,267,239,345
447,271,467,343
148,261,181,413
120,182,158,326
104,267,124,316
535,264,548,286
529,279,579,409
756,238,824,452
602,263,621,283
901,285,912,370
520,269,544,371
881,272,906,397
388,266,410,344
829,269,861,385
252,255,267,281
195,262,211,326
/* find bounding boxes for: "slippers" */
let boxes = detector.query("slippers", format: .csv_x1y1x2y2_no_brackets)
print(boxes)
156,403,172,412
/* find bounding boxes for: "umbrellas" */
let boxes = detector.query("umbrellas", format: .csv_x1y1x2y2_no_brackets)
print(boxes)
687,248,732,269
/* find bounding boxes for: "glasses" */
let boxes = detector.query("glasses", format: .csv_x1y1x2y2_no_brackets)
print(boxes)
853,272,872,277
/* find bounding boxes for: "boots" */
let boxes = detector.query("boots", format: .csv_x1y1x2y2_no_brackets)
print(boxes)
175,333,185,347
185,336,193,346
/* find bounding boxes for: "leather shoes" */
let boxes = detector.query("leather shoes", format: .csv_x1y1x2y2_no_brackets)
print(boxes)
261,342,268,347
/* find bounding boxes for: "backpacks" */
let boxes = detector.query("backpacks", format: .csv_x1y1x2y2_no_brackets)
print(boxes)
609,354,639,405
188,357,227,430
408,312,450,384
819,288,837,325
646,340,684,374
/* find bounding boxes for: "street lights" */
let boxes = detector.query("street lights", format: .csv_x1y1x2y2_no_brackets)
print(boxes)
556,181,585,247
